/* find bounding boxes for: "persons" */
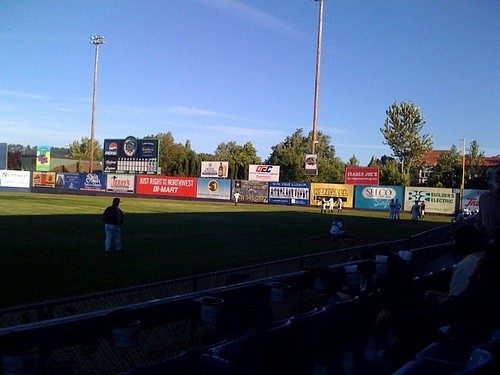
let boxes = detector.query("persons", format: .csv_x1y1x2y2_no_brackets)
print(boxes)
389,199,396,220
464,169,492,190
439,224,484,304
475,182,500,243
234,191,239,206
443,173,460,188
320,196,342,214
410,201,425,222
330,216,345,240
394,199,401,220
417,178,445,188
102,198,124,258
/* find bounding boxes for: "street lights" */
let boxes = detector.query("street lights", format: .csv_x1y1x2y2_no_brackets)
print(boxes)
90,33,105,173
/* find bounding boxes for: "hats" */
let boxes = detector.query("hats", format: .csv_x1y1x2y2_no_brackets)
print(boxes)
114,199,120,204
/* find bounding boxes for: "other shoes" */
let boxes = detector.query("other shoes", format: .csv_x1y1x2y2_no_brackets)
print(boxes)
118,249,124,256
104,250,110,257
